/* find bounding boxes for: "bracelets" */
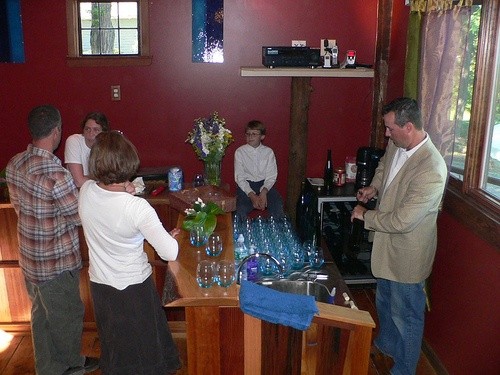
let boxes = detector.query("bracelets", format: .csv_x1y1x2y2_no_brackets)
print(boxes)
360,209,368,224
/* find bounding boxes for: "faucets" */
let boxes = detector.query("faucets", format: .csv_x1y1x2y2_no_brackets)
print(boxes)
236,252,284,286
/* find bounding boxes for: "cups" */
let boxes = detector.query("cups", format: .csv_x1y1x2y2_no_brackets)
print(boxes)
232,212,325,270
196,260,214,288
204,234,223,256
216,260,236,288
189,224,207,247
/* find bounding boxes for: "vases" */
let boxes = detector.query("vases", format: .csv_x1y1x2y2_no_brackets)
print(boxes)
199,155,225,186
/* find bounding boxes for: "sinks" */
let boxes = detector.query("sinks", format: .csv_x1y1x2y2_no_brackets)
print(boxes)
252,279,338,306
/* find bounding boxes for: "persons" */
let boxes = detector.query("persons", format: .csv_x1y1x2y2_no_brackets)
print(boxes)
350,95,449,373
76,129,184,371
64,113,108,193
6,104,100,372
234,120,285,219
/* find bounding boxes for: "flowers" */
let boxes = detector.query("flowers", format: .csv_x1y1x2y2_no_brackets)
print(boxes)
183,196,225,237
183,109,235,160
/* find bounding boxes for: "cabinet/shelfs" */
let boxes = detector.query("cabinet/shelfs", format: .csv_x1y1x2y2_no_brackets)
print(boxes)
302,178,379,285
240,65,375,80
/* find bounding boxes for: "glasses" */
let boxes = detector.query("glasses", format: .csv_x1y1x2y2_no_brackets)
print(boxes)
96,130,123,139
244,132,262,137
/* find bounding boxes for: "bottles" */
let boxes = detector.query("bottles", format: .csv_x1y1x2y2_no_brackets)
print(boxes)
325,149,333,182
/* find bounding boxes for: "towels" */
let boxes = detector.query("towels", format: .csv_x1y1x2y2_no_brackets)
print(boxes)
239,281,320,332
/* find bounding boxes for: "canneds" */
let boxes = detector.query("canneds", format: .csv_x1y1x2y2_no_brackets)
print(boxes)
168,167,183,193
332,167,345,186
